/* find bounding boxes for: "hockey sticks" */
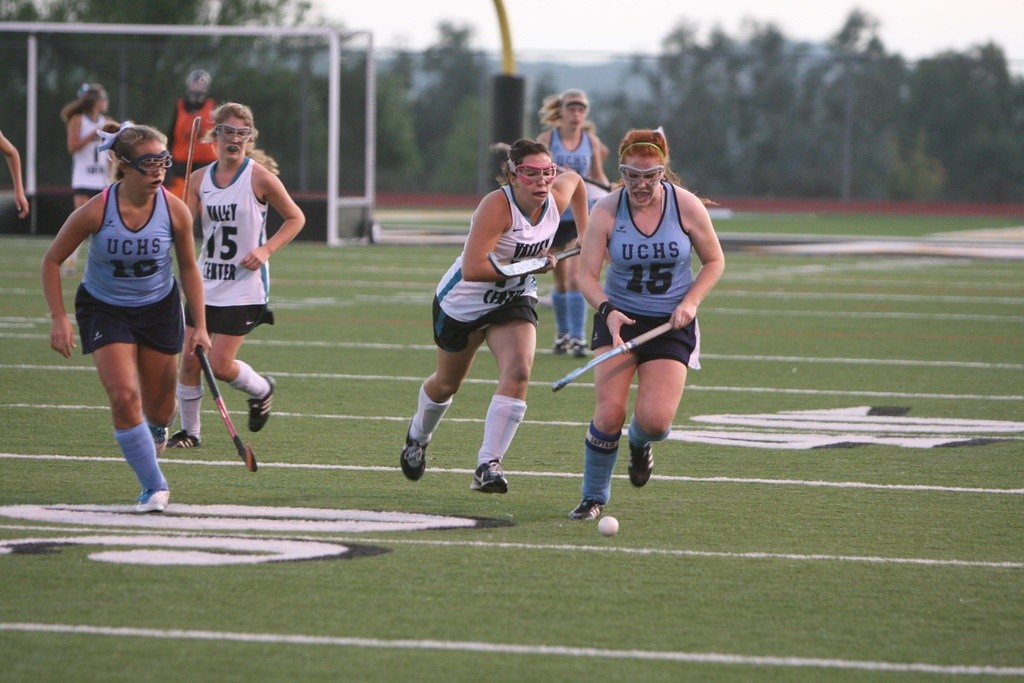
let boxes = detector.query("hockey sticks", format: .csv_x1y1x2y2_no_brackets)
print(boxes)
195,343,259,473
182,115,202,206
486,246,582,279
551,322,673,394
494,141,613,192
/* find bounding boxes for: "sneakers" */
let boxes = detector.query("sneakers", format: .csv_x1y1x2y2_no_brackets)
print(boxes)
400,420,430,482
567,498,604,523
144,419,168,455
164,430,200,451
135,488,170,514
627,440,654,488
248,377,275,431
467,456,507,494
553,334,569,355
568,338,589,358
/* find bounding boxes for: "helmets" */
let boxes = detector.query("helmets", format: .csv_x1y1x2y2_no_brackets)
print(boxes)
183,69,212,107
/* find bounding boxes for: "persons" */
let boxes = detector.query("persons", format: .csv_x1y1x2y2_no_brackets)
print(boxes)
535,86,626,359
0,128,28,219
60,83,121,276
399,138,590,493
566,125,727,520
42,124,211,515
168,103,307,447
168,66,221,219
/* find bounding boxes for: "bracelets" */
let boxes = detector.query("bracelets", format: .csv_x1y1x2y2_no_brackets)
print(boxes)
599,301,619,323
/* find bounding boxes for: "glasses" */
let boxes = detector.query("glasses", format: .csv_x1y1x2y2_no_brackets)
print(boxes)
210,123,253,142
130,150,172,176
619,165,664,183
516,164,557,181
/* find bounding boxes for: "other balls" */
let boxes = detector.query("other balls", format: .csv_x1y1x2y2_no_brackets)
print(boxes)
598,516,620,536
